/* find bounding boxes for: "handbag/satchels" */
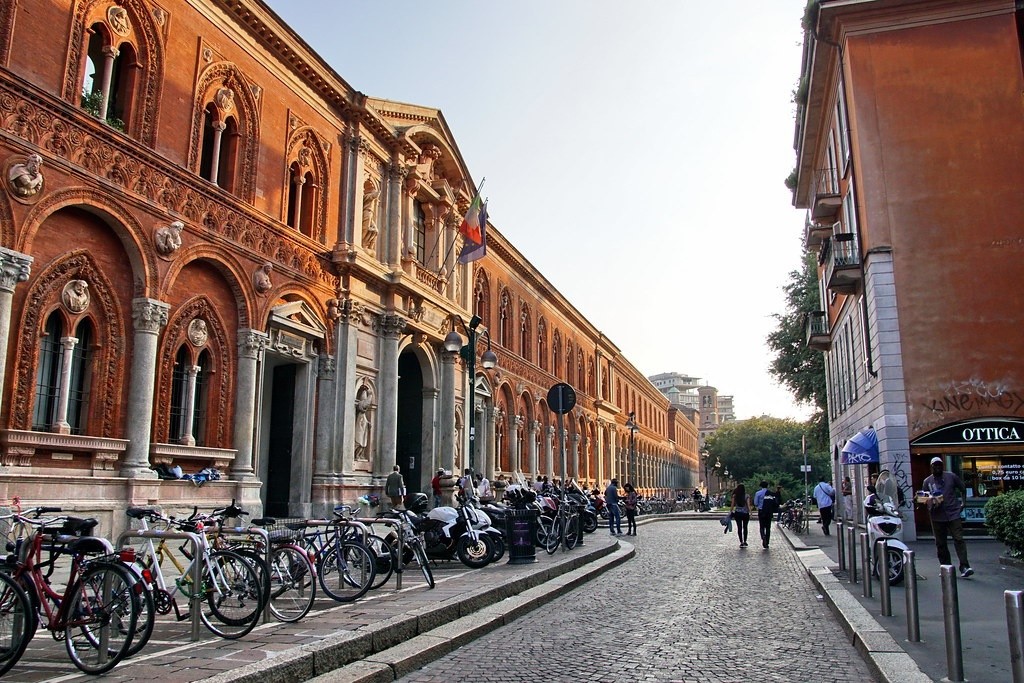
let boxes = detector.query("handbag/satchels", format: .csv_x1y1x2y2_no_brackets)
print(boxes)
626,532,631,535
830,495,835,503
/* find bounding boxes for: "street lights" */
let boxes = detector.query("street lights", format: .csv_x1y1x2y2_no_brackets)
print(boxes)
443,310,499,483
723,465,730,491
622,411,637,488
701,449,712,510
715,455,721,494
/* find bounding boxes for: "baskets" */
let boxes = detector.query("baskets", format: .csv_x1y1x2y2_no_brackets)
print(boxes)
265,517,307,543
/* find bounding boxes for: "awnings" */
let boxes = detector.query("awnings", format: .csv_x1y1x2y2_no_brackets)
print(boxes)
840,429,879,464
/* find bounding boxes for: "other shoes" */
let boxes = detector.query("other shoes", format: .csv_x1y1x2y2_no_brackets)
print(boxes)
764,546,769,549
822,526,829,535
762,535,767,546
817,519,821,523
618,531,622,533
611,531,615,534
631,532,636,536
961,567,974,577
740,542,748,547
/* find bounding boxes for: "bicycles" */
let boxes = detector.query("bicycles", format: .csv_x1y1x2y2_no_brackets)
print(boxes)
777,494,813,535
0,476,695,673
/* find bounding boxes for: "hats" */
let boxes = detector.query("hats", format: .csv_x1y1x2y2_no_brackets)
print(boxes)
435,468,446,476
930,456,943,465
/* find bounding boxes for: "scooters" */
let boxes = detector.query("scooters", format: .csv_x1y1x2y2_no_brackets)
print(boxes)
862,470,911,586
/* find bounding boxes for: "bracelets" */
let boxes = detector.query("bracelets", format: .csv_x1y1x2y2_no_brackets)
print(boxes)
960,505,962,506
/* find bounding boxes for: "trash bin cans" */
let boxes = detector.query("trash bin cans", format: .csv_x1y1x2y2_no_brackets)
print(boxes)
505,509,539,564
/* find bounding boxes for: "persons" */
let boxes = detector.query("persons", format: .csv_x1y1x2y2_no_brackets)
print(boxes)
432,471,443,507
255,262,273,291
605,479,637,536
813,470,895,534
730,484,752,547
191,321,207,344
922,457,974,577
362,179,380,247
455,417,459,458
534,475,574,492
12,154,42,188
65,280,88,311
355,389,373,458
465,469,513,496
678,491,685,500
385,465,404,506
155,222,183,251
694,488,701,511
968,509,984,518
754,481,776,549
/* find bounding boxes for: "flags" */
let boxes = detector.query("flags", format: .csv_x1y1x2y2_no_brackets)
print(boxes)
457,194,489,264
